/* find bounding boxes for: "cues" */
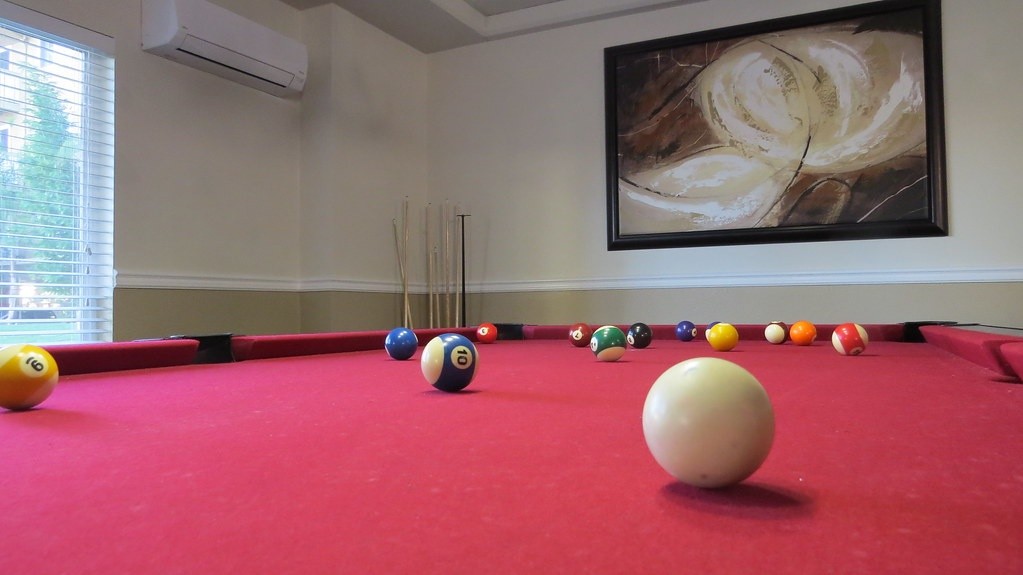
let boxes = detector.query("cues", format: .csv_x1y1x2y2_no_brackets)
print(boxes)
404,195,409,328
456,214,472,327
428,202,434,329
446,218,450,328
455,218,460,328
434,246,441,328
392,218,414,330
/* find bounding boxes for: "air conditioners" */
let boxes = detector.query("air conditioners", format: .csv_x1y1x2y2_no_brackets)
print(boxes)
139,0,309,99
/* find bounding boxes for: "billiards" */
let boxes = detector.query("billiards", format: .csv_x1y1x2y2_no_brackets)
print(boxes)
568,322,592,347
788,320,817,346
420,333,479,393
590,324,627,361
626,322,653,349
704,321,721,342
384,327,418,360
708,322,739,352
641,356,775,490
831,323,868,356
476,322,497,343
763,320,788,344
0,344,60,411
675,321,697,342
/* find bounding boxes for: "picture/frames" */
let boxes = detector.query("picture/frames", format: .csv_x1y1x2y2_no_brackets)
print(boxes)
602,1,951,253
